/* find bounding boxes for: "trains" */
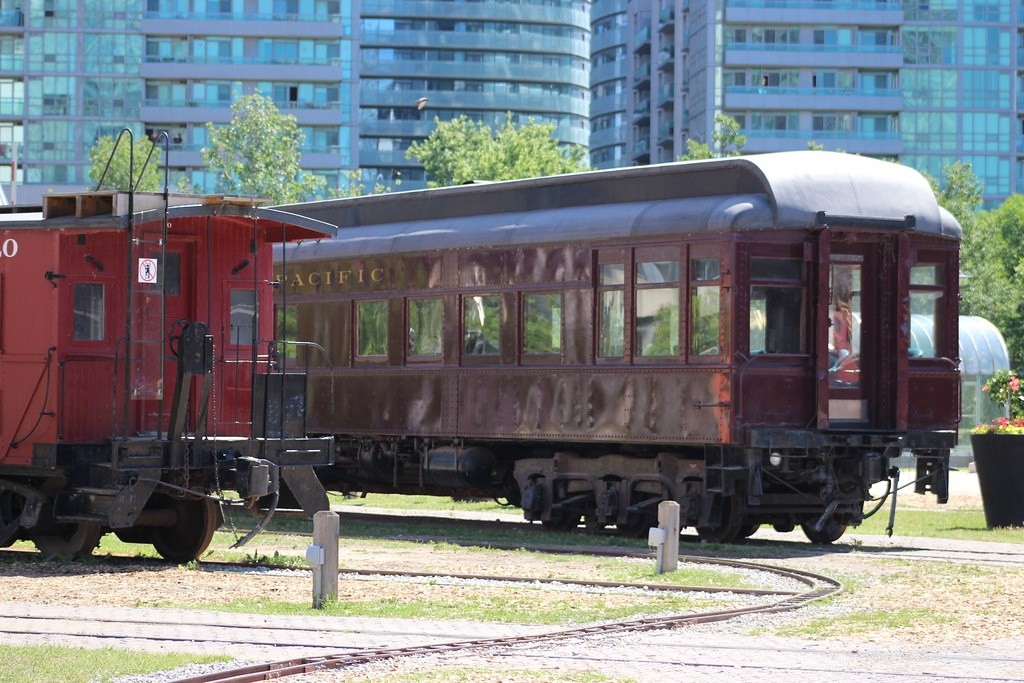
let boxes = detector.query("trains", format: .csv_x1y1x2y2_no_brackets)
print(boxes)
0,125,341,560
267,147,962,550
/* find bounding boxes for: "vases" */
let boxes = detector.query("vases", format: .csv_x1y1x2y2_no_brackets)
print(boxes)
970,435,1024,531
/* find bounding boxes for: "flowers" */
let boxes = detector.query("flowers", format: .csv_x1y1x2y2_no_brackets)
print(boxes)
970,367,1024,434
